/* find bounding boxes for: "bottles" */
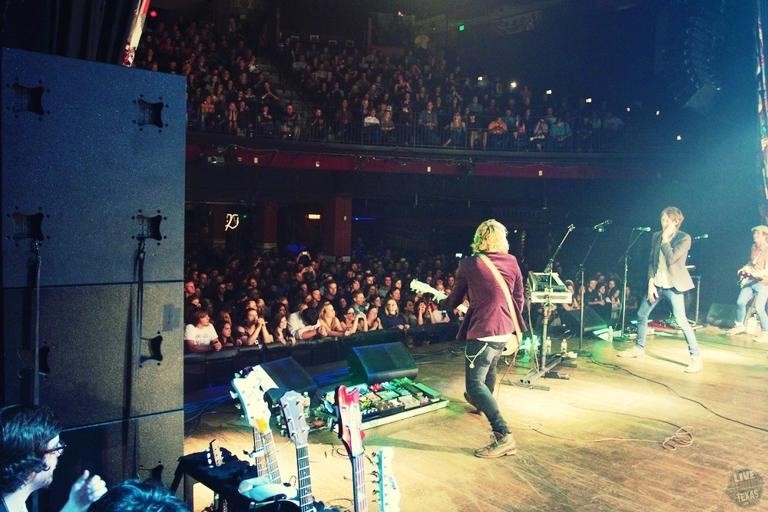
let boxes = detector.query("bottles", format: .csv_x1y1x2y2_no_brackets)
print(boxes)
608,326,613,336
561,338,567,353
524,336,531,353
546,336,551,353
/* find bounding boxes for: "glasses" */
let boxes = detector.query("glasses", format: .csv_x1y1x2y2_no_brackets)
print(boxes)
38,437,70,457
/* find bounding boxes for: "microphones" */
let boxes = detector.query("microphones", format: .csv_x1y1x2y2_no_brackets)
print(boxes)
693,233,709,240
632,225,656,232
592,218,613,231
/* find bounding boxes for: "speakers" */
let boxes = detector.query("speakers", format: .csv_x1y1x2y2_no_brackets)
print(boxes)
0,46,188,512
705,302,738,328
347,341,419,386
559,306,609,337
260,356,319,415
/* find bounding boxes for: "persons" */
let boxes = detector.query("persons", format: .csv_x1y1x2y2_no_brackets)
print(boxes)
0,403,109,512
431,219,526,461
723,224,768,345
616,204,705,374
183,246,615,351
133,12,635,153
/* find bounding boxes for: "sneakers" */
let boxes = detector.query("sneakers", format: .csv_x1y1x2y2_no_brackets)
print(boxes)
616,346,645,359
474,431,515,457
753,335,768,343
724,325,745,336
684,360,705,372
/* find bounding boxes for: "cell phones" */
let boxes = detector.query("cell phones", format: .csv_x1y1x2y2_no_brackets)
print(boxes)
355,309,360,315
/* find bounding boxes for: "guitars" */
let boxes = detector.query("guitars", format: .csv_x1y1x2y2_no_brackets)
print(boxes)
206,368,401,512
410,279,523,356
739,264,768,288
